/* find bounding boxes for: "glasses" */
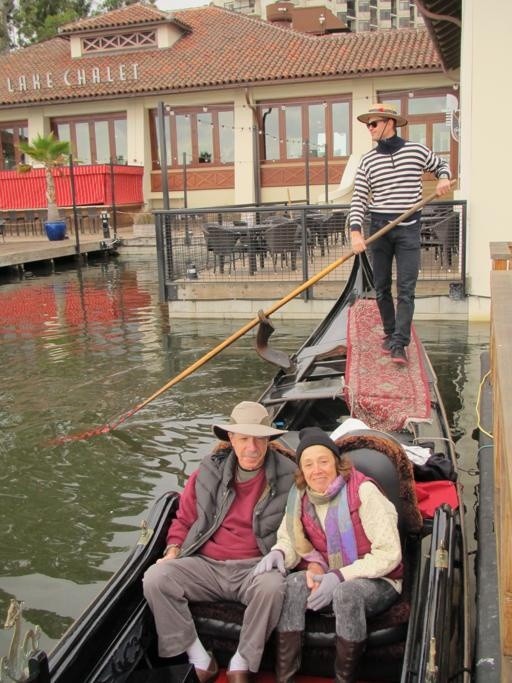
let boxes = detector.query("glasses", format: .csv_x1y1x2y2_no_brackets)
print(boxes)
366,118,388,129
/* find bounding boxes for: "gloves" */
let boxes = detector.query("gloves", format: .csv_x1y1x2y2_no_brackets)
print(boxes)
306,568,346,612
253,550,287,575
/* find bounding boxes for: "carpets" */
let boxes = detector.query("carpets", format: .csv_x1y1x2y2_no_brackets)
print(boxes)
344,291,432,434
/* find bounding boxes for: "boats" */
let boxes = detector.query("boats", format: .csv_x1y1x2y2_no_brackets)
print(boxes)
18,239,470,682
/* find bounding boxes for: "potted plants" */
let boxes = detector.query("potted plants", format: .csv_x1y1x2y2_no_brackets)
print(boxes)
15,132,83,242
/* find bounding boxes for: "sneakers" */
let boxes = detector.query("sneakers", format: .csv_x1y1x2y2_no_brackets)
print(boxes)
390,343,409,366
381,334,393,355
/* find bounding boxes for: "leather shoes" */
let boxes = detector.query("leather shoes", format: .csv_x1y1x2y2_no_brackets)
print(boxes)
195,650,219,683
225,660,250,683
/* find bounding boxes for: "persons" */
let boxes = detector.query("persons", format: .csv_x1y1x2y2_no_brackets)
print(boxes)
141,401,302,683
100,209,112,239
253,426,404,683
346,102,453,365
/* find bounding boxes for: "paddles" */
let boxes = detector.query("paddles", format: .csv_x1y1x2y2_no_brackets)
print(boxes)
48,180,460,446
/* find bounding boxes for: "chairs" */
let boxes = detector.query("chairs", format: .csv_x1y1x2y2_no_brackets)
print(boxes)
298,429,422,668
200,200,463,273
172,444,298,657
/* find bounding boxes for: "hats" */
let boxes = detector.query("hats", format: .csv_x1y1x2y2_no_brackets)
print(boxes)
212,401,289,443
357,103,408,128
296,426,341,468
